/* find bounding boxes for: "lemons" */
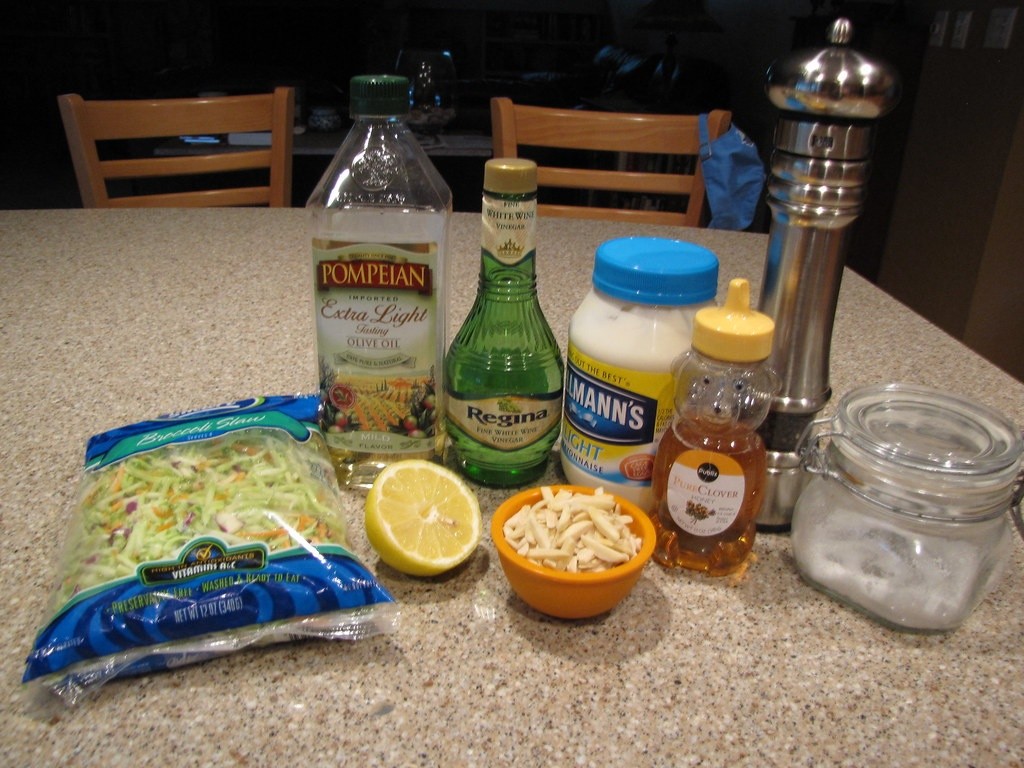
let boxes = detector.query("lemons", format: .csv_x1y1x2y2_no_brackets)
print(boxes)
365,459,483,576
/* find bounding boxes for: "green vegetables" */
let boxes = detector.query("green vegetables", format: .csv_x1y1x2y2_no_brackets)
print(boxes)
55,433,337,620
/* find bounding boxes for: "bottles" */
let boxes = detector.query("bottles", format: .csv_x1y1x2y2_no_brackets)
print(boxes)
304,74,453,489
563,237,721,514
651,276,776,575
790,383,1024,630
443,157,564,489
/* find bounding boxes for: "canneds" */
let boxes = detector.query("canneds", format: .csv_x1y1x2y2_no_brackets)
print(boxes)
559,234,718,514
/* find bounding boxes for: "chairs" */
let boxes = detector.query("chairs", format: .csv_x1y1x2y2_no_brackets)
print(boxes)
489,96,732,229
56,89,292,208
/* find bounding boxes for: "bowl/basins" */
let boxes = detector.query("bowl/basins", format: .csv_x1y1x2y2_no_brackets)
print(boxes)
492,485,656,620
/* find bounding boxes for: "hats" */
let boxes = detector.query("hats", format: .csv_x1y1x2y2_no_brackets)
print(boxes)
699,113,766,230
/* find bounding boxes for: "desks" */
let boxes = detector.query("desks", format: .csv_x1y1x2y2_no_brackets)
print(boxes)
0,208,1024,768
148,128,493,163
